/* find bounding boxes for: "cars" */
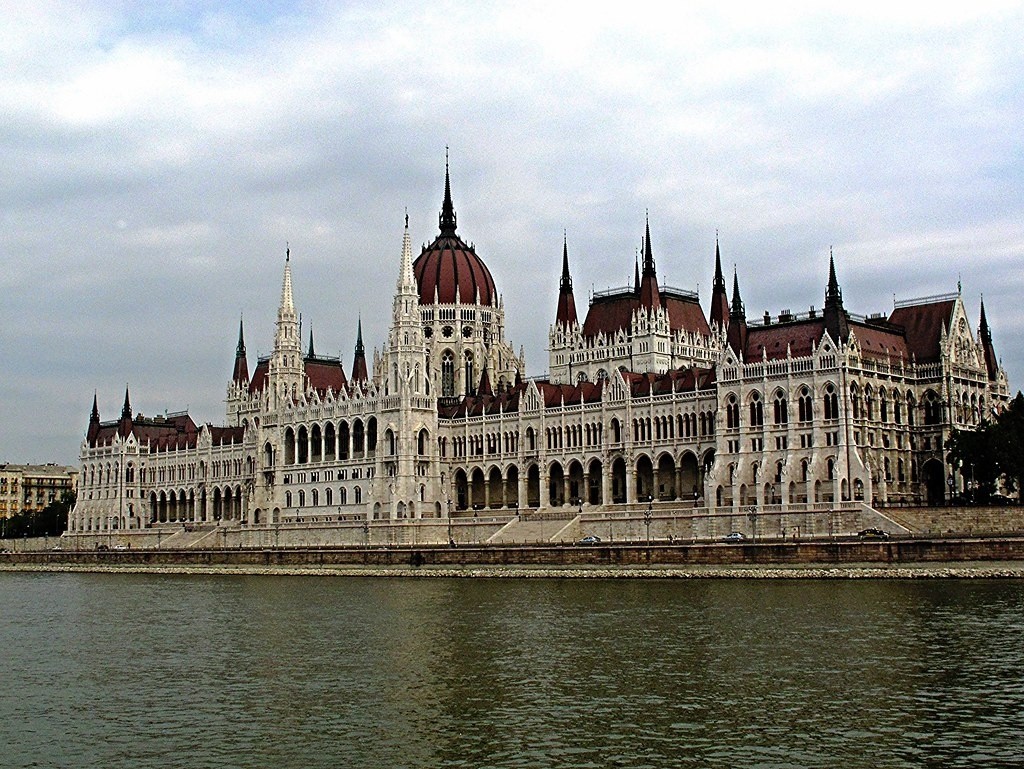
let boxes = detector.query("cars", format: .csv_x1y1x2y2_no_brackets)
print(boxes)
113,544,128,549
723,532,748,539
579,536,601,542
858,528,890,540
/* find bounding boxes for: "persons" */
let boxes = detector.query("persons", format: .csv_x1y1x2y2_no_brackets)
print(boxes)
449,537,456,548
94,540,99,551
127,542,131,551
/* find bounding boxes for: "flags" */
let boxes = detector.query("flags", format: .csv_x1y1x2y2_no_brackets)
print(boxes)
973,404,981,418
992,404,1000,420
